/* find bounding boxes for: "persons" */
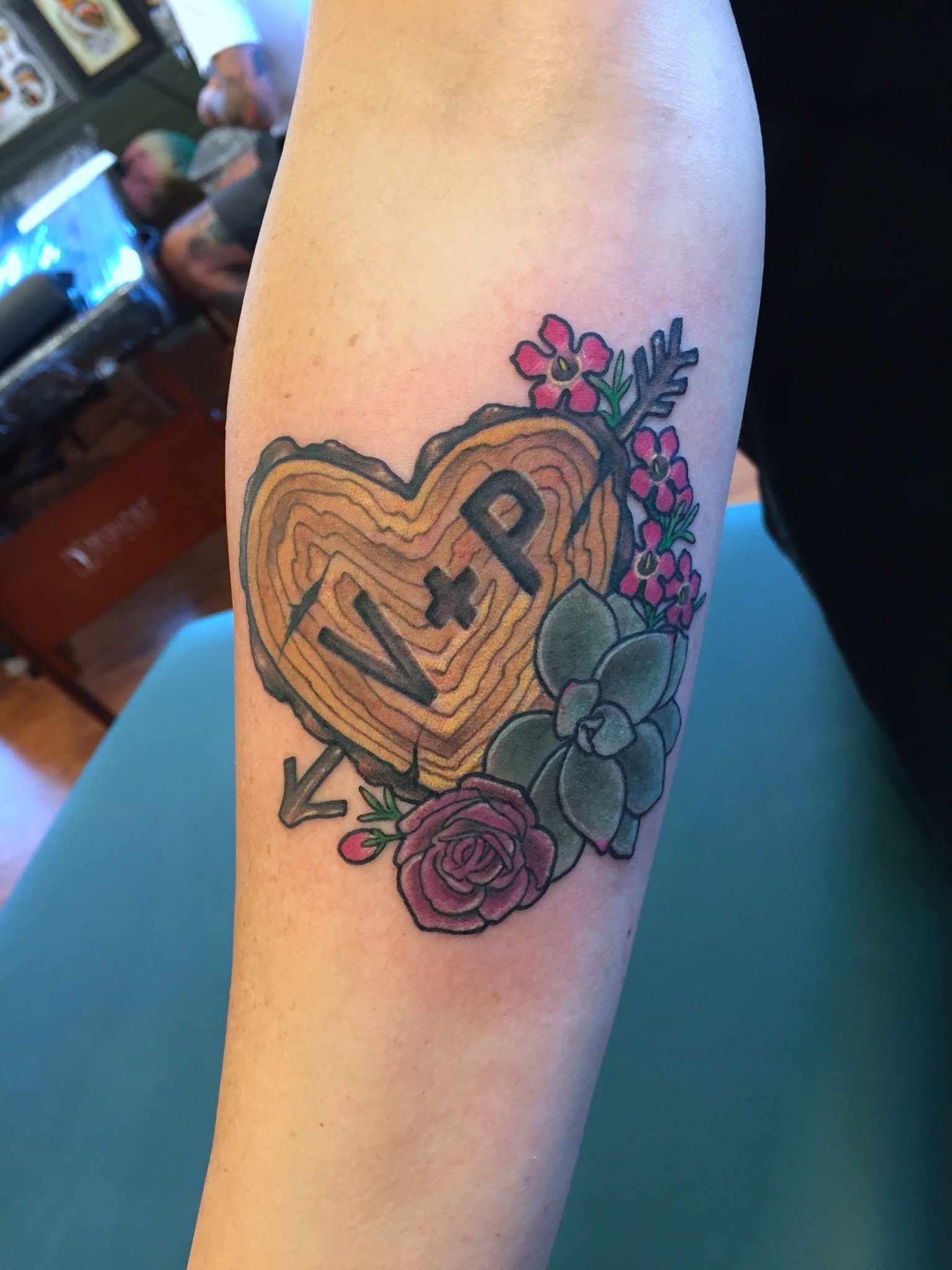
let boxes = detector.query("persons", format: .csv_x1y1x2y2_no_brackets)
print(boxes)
180,0,768,1269
155,1,313,347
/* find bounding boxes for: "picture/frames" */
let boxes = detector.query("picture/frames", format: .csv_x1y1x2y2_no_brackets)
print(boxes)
17,0,169,91
0,0,80,150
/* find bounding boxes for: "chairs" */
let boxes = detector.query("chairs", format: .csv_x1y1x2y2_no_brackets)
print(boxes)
0,277,238,734
